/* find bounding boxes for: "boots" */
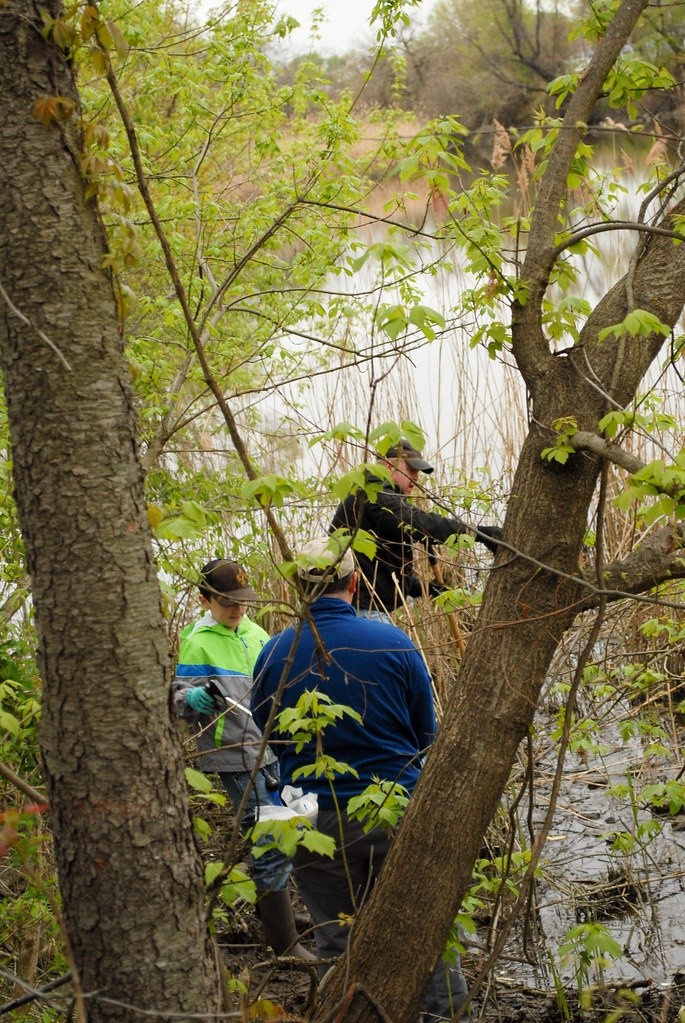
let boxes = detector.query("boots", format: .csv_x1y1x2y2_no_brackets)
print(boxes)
254,888,317,961
292,908,310,928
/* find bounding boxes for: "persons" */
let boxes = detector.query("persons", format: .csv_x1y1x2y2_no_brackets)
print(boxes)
250,535,474,1023
328,439,502,618
174,558,322,971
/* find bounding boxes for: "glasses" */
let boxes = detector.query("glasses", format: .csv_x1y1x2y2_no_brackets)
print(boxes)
209,592,245,607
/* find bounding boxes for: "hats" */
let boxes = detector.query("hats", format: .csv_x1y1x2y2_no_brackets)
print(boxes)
297,537,354,583
376,439,434,474
200,558,259,602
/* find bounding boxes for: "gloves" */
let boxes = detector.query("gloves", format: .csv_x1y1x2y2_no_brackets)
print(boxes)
475,526,504,555
186,686,214,714
